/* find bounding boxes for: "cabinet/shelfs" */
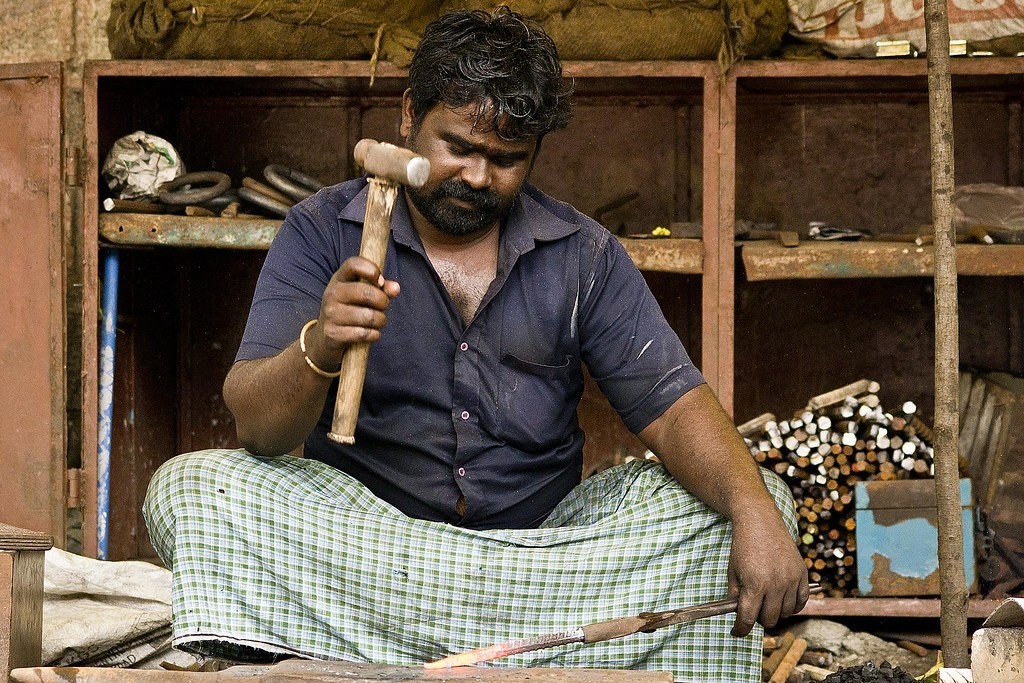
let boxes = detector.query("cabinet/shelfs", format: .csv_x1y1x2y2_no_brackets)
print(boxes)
1,60,1024,619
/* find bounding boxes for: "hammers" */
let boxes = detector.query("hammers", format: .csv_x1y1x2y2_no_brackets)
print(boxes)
326,137,431,443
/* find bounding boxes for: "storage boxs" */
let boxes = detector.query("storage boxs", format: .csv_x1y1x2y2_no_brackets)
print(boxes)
855,477,980,597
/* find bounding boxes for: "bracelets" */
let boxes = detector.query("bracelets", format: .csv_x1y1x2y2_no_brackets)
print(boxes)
300,319,341,377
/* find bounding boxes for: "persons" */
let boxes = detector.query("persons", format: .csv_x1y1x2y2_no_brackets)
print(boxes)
142,5,811,683
